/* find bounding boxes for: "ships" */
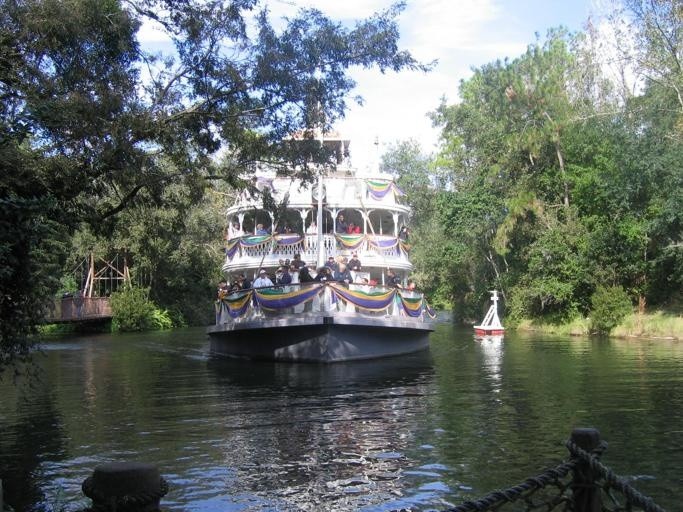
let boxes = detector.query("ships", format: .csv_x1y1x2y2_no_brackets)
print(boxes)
206,128,437,365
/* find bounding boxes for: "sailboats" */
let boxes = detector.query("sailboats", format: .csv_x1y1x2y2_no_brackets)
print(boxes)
475,335,505,403
473,289,506,337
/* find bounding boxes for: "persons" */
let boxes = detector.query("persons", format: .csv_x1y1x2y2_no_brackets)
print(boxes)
217,253,416,300
61,289,83,317
222,214,361,241
399,225,410,241
